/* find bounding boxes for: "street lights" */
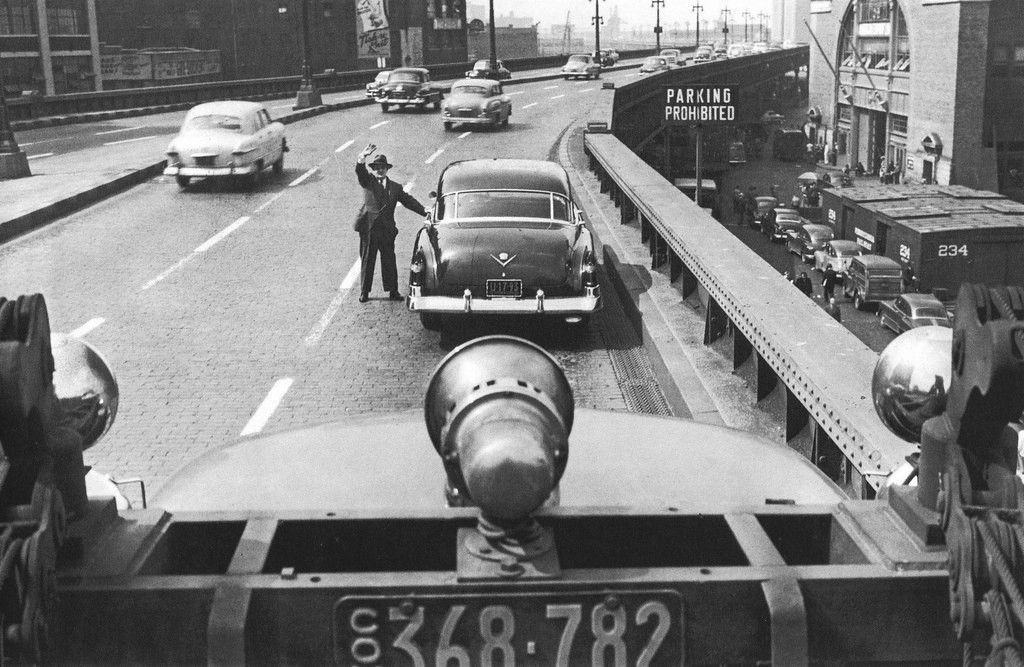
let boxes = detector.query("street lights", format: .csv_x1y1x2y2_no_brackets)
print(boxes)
589,0,606,63
651,0,665,56
741,8,750,42
756,10,769,42
721,5,732,44
691,0,704,51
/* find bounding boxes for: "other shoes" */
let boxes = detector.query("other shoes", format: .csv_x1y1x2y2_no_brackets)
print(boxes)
389,291,404,301
359,292,368,303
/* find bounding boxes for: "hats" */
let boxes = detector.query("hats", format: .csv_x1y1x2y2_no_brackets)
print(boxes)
368,154,393,169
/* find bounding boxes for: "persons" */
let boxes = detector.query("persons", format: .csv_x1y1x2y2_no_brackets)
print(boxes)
888,160,900,173
823,297,840,323
733,184,756,225
824,142,829,164
806,142,813,153
770,185,778,198
802,184,819,207
823,262,836,301
794,272,813,298
878,156,885,182
352,143,428,302
845,161,864,174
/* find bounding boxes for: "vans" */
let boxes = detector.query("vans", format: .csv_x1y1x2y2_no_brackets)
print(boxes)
673,176,719,222
774,127,805,163
728,142,747,167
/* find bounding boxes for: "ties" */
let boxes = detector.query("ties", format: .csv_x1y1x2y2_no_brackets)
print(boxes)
380,178,384,189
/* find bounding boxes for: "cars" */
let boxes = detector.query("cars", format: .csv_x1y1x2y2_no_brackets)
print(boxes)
591,48,619,68
817,172,855,190
693,39,799,63
761,109,786,126
163,101,291,191
786,224,836,264
559,55,601,81
813,239,864,284
442,78,512,132
841,254,905,310
761,208,802,241
638,49,686,76
406,157,604,346
365,69,392,98
876,293,955,336
749,197,778,230
465,59,511,81
374,67,445,114
